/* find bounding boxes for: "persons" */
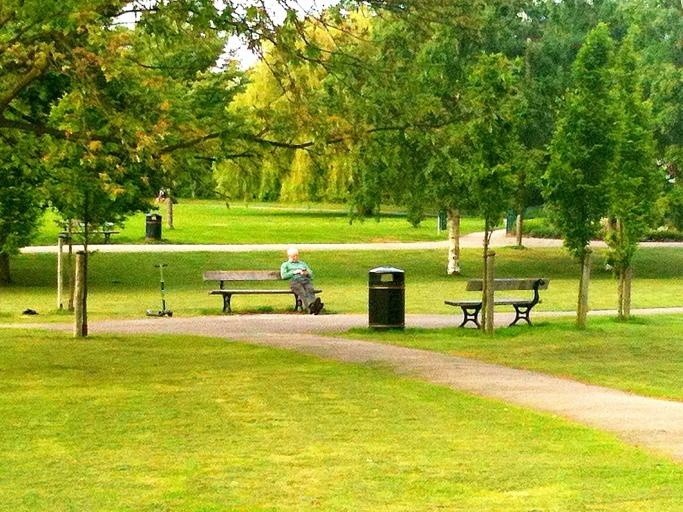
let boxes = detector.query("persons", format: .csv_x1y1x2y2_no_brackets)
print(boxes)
157,188,167,203
280,247,324,315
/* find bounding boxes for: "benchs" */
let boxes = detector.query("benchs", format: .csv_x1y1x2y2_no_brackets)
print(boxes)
203,270,322,316
444,280,548,329
62,222,120,244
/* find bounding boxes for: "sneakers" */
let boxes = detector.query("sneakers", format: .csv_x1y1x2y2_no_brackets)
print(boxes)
309,297,324,315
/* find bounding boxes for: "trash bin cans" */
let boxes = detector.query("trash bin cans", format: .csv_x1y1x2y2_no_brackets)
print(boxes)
369,266,404,329
146,214,162,240
